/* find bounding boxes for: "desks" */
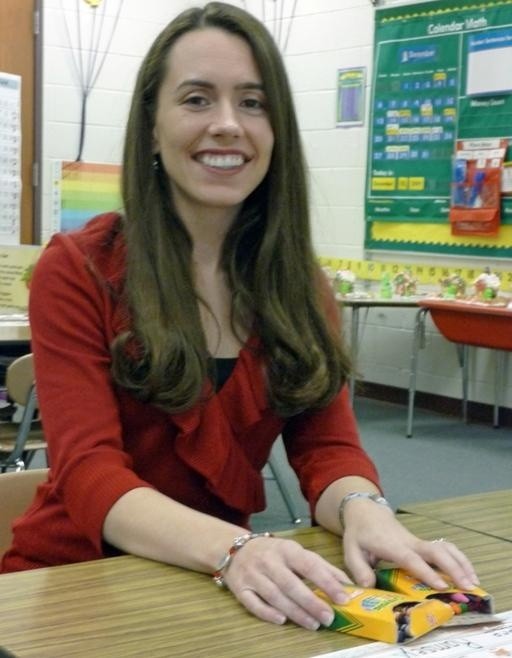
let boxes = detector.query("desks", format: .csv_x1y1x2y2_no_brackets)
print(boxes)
337,297,469,429
396,487,511,542
0,512,512,658
0,320,33,342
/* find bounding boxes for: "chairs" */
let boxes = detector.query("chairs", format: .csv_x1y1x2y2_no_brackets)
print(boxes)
0,352,43,475
0,468,50,561
406,299,512,438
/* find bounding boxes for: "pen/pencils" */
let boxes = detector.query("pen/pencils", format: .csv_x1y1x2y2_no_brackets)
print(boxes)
393,593,493,643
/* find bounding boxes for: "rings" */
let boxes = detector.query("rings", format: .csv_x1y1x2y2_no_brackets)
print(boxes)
429,535,445,544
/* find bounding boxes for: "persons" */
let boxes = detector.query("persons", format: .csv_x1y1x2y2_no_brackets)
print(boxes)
0,0,485,634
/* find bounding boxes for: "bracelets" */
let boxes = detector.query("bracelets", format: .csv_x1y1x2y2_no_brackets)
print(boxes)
212,531,273,590
338,490,395,532
